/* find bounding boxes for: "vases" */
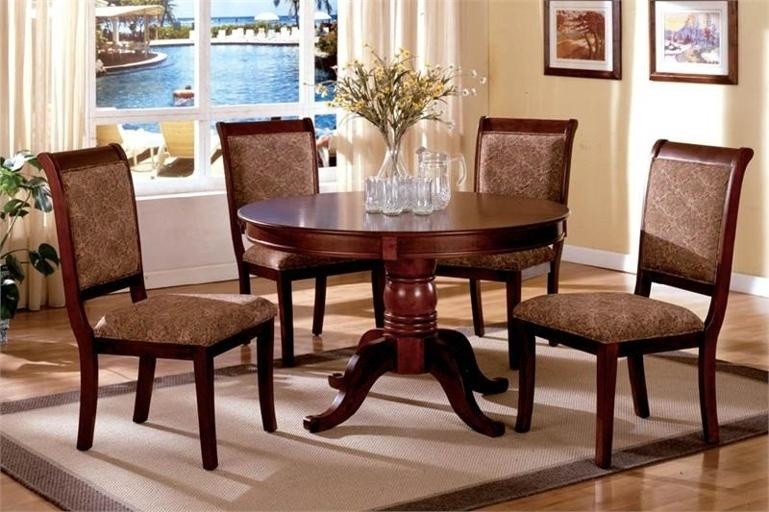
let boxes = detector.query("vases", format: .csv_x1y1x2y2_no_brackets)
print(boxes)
301,37,486,174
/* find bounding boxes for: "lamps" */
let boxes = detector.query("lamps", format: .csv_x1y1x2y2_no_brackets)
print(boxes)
319,133,337,168
188,26,297,44
36,143,278,471
95,106,166,167
507,139,753,468
152,116,220,178
435,115,578,369
216,119,386,364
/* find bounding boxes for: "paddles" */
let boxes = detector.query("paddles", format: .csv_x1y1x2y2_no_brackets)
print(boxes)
0,322,769,512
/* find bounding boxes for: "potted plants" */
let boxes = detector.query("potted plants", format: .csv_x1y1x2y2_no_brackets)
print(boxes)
648,0,738,86
543,0,621,79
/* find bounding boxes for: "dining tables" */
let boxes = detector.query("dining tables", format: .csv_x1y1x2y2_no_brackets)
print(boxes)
0,150,60,343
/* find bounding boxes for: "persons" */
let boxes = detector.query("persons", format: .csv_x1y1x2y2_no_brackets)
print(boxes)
314,124,339,167
171,84,194,107
96,58,108,76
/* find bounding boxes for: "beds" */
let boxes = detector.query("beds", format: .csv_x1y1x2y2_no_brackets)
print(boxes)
254,10,280,34
312,11,333,21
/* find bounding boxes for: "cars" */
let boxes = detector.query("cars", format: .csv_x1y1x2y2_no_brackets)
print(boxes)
363,174,435,216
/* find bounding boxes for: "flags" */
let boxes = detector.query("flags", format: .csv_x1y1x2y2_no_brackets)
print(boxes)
377,150,408,178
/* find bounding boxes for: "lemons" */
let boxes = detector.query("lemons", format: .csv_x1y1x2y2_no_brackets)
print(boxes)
413,151,469,213
